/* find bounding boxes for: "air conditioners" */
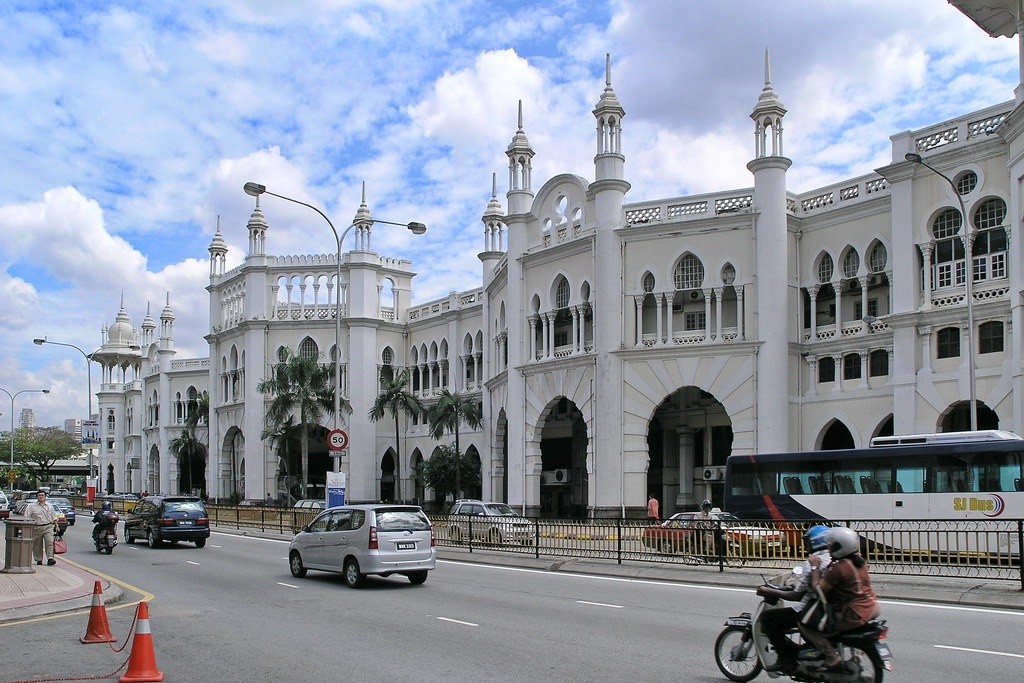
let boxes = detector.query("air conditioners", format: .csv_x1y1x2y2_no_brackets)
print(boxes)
869,275,881,285
586,307,591,314
848,279,860,289
703,467,721,480
556,470,571,482
564,309,571,317
673,304,683,312
689,291,704,301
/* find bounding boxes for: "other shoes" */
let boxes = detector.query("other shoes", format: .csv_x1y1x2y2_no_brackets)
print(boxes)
48,559,56,565
766,663,793,672
37,561,42,565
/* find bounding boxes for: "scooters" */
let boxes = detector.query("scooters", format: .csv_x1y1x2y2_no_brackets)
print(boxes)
89,509,120,555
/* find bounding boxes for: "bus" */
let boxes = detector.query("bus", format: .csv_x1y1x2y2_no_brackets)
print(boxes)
725,430,1024,564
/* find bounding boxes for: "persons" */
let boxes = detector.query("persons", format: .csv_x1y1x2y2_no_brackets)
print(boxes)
182,493,187,496
693,499,725,553
92,501,114,545
12,490,17,499
647,493,659,525
756,525,880,672
24,491,60,566
266,493,273,502
143,491,150,497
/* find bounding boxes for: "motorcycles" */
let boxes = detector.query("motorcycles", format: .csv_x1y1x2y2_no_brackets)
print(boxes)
713,566,894,683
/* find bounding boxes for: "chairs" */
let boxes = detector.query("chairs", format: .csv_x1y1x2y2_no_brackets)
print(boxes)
958,479,966,491
887,481,903,493
988,470,1002,492
336,518,350,530
860,476,881,493
809,476,829,493
783,477,804,495
834,475,855,493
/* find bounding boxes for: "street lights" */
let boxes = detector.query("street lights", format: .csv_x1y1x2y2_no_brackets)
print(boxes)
0,388,50,493
243,182,427,510
33,338,141,515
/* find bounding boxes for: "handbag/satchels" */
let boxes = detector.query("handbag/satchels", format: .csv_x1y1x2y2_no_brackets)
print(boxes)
798,597,836,634
53,532,67,554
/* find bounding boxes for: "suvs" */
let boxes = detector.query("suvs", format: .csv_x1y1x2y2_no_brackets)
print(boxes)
124,494,210,549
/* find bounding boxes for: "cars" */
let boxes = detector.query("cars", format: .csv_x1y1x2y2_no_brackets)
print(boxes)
448,499,536,545
289,504,436,589
293,499,325,509
23,486,139,503
642,512,785,569
45,498,75,525
0,492,9,520
238,499,267,508
13,504,68,536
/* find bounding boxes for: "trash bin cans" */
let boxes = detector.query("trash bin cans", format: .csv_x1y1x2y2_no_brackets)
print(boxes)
0,517,37,574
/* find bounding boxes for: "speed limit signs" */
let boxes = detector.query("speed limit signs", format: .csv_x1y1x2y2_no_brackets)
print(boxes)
327,430,348,452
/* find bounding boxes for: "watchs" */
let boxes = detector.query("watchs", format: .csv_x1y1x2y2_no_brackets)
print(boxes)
811,566,819,570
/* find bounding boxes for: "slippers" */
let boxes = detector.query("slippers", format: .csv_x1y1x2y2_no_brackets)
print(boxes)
816,664,855,674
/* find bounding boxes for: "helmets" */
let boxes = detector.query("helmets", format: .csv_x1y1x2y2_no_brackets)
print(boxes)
102,501,111,509
825,527,860,559
800,525,830,553
701,500,712,506
